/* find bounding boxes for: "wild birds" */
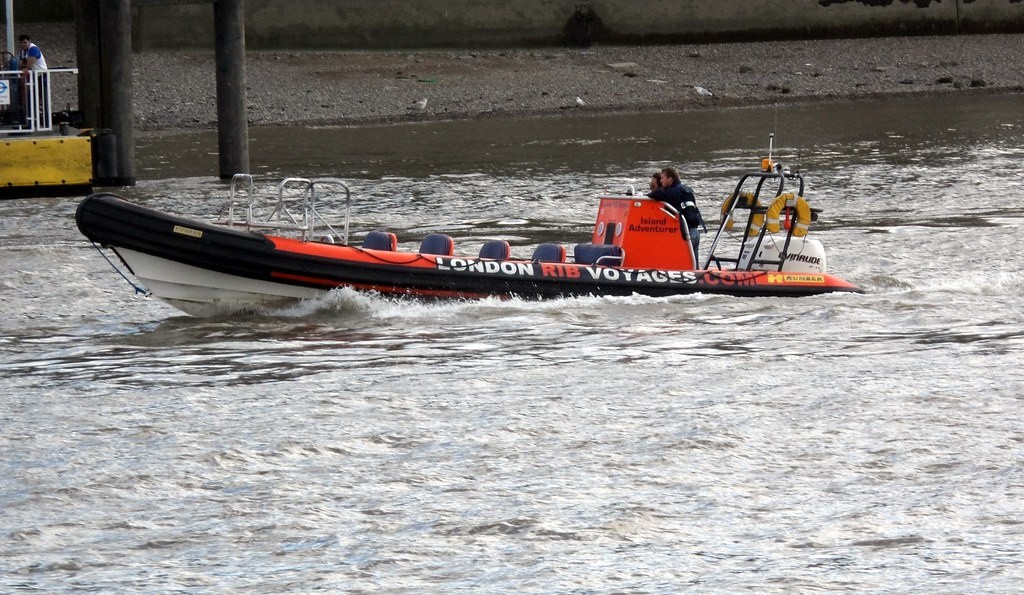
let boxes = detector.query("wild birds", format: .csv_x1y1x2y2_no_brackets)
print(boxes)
690,85,712,100
575,96,591,111
404,98,428,115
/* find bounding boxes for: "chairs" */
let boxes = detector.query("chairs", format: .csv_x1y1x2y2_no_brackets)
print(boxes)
364,231,626,268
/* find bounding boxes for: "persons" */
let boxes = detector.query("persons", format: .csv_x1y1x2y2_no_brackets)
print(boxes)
2,52,19,70
648,167,707,269
18,35,48,130
649,174,664,191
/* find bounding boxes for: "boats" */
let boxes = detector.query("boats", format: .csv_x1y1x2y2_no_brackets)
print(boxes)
69,99,866,321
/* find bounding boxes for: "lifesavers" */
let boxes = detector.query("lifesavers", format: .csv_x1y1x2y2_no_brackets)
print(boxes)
719,192,764,237
766,193,810,236
